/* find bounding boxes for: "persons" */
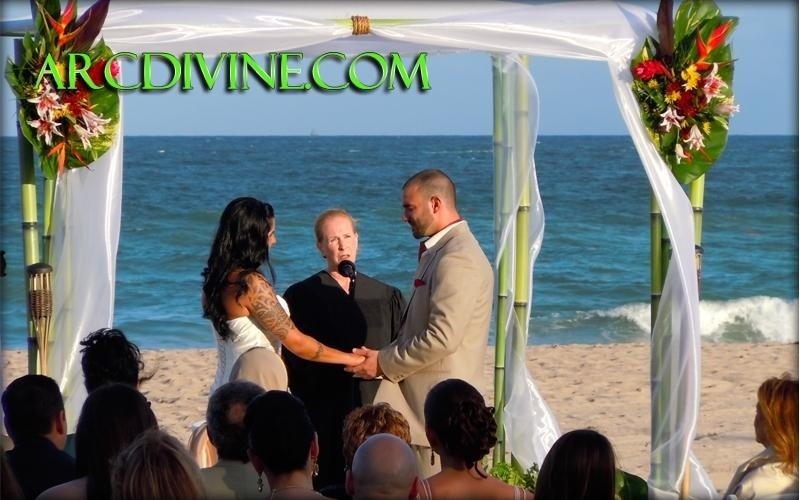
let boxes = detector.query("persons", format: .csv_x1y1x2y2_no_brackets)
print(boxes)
35,385,159,500
346,434,420,500
318,403,418,500
243,390,336,500
2,376,77,500
344,169,493,480
533,430,616,500
198,382,271,500
281,211,408,438
201,197,365,399
722,372,800,500
61,329,145,482
111,423,206,500
420,379,535,500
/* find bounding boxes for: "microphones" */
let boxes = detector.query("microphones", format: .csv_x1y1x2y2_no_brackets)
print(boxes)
338,260,357,279
